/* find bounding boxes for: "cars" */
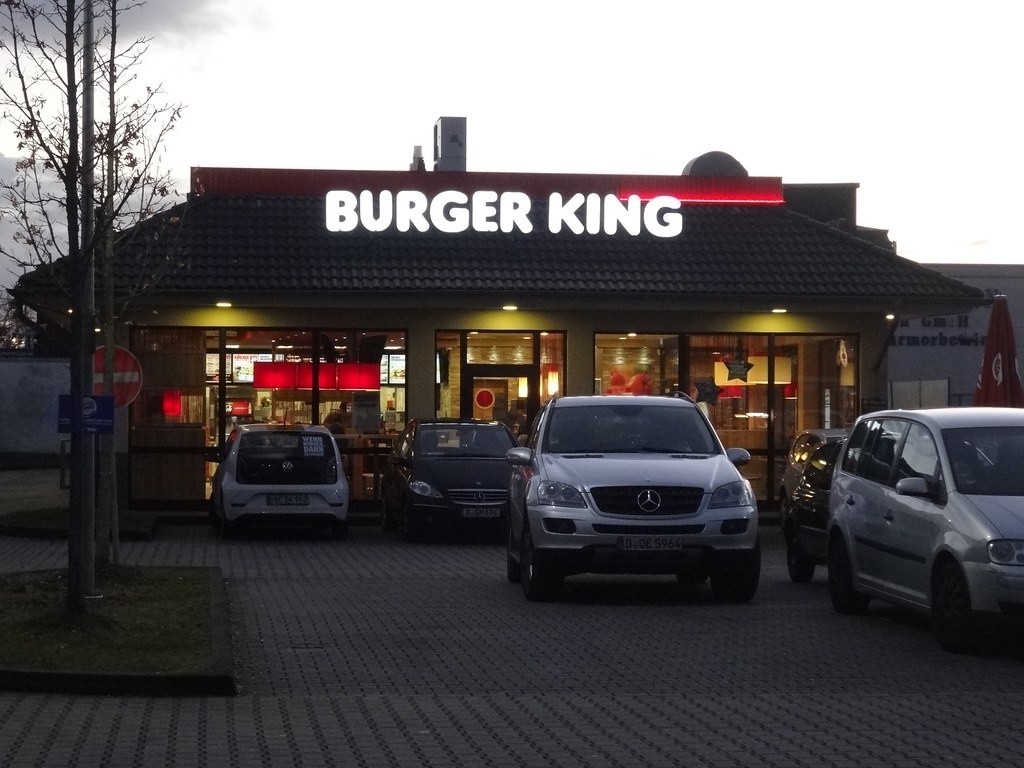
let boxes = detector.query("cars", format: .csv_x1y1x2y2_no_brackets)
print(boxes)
777,430,857,581
825,407,1024,651
377,416,522,539
203,422,349,536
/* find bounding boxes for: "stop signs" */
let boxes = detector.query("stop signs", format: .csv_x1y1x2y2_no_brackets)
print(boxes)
91,343,143,413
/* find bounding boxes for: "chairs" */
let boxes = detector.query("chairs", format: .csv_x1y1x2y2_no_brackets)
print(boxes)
470,430,505,457
419,432,444,454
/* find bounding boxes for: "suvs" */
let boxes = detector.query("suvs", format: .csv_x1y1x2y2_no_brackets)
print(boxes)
501,388,763,605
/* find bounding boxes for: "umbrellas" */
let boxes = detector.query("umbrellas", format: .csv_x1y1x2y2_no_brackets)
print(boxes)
969,295,1024,408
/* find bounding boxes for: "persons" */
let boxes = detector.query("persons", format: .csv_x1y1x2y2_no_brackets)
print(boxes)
323,402,350,453
501,402,527,434
